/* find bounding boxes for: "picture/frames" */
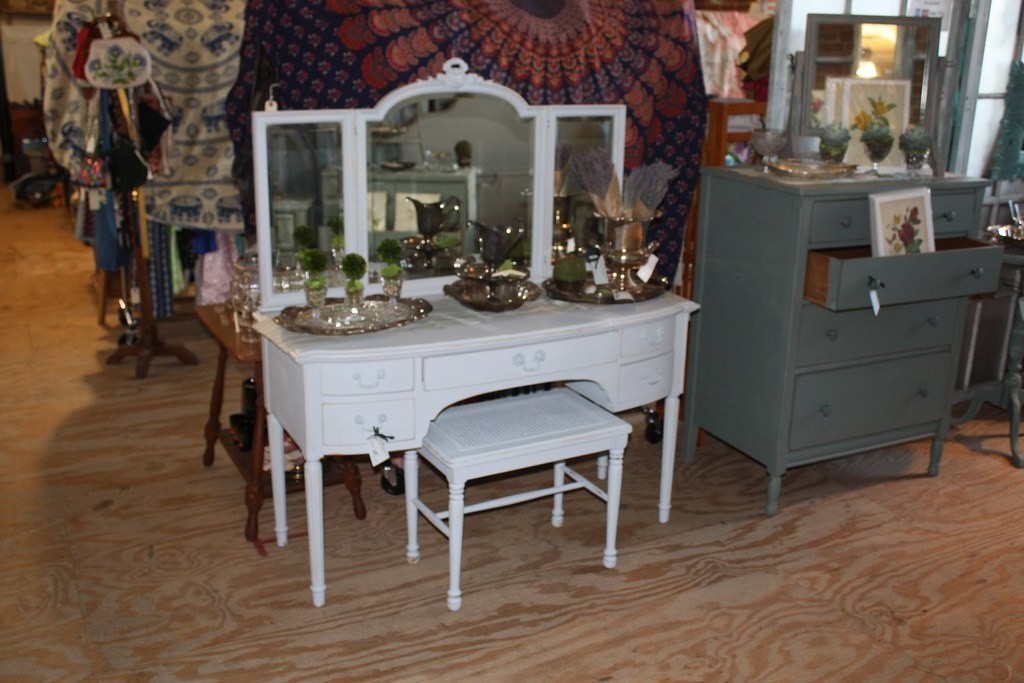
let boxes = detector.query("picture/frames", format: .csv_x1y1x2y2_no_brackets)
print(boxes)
840,78,912,168
869,186,936,257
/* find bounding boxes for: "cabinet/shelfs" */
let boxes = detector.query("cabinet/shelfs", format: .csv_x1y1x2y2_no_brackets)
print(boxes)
681,167,1006,517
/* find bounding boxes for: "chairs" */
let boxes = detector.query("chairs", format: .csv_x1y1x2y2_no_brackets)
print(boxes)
671,99,769,448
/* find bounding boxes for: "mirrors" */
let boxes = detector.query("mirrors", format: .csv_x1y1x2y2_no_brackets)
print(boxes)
551,112,617,266
799,13,936,140
266,122,349,292
368,91,538,282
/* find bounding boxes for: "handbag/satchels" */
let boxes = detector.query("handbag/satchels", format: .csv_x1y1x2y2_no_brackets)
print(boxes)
81,16,171,190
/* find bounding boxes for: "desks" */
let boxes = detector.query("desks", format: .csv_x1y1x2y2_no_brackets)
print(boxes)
250,277,701,609
407,387,633,612
193,303,366,539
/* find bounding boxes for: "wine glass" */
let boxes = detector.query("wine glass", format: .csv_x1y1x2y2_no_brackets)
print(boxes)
750,129,788,174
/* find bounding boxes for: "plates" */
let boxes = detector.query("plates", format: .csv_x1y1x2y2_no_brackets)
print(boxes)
767,158,859,180
986,224,1024,245
